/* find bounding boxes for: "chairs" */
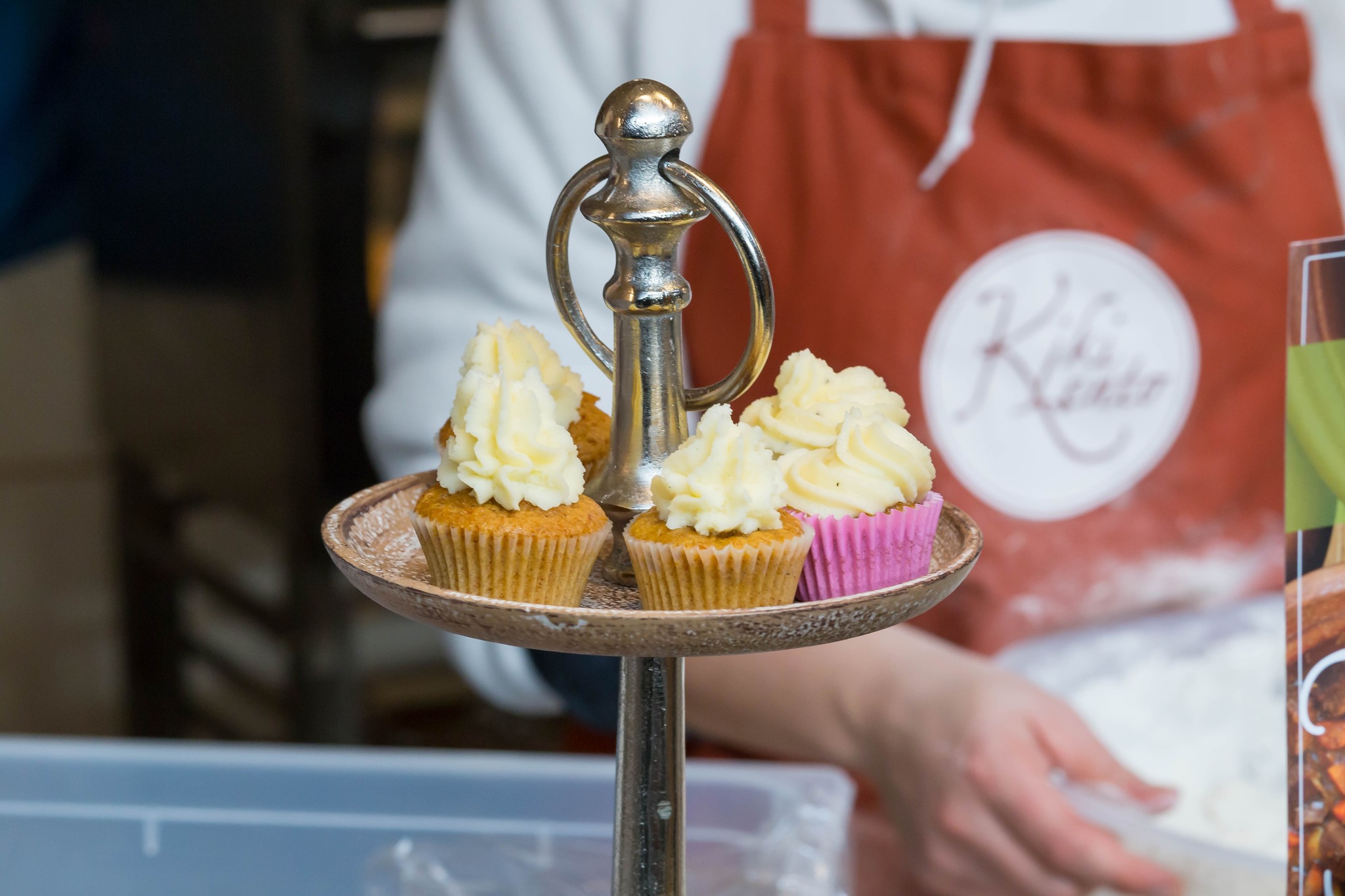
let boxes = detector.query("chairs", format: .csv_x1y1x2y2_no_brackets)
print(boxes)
117,446,552,751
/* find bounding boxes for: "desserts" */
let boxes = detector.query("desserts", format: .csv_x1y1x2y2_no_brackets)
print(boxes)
412,321,942,612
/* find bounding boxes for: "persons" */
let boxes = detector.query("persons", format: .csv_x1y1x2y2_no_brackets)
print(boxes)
363,0,1344,896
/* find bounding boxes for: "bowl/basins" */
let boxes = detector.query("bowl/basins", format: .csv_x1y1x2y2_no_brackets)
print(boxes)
993,601,1288,896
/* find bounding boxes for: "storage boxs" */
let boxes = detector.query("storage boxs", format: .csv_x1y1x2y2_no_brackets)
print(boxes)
0,733,856,895
